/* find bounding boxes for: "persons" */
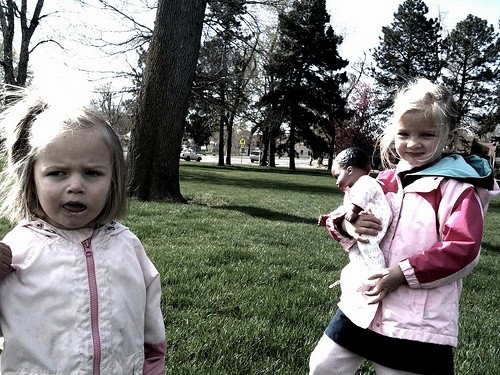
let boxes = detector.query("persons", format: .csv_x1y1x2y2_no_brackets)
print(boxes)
308,79,499,375
0,81,165,374
332,147,393,292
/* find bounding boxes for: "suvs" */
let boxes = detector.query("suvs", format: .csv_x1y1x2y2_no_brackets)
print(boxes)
249,150,269,164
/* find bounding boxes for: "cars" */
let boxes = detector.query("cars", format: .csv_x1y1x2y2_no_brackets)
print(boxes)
180,149,202,162
